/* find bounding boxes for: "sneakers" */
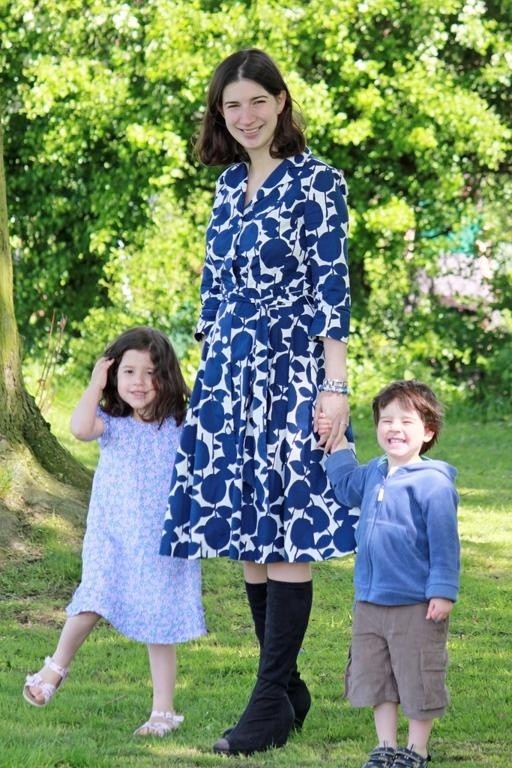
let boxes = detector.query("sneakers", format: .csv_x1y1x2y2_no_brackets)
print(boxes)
364,746,394,767
392,748,426,767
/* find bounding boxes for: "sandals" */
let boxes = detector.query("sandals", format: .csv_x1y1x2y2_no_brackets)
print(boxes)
134,710,185,738
22,656,65,709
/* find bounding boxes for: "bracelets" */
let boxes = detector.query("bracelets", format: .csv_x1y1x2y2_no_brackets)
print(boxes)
322,377,349,394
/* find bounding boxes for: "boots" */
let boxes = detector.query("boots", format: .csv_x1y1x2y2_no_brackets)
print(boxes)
214,580,312,753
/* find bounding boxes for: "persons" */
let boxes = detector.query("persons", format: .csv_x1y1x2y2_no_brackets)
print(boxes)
21,328,207,735
310,380,461,767
158,50,355,752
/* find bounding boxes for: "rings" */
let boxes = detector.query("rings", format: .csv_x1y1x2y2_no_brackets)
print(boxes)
341,422,347,425
